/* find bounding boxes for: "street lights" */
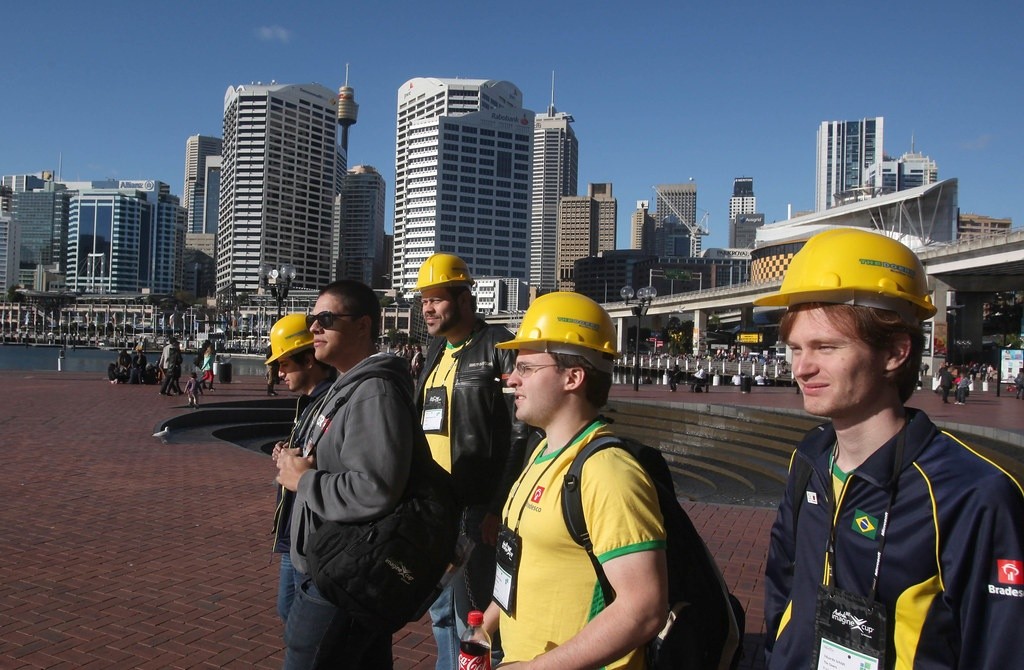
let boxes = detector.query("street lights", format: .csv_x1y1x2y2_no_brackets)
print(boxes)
621,283,655,393
267,264,298,321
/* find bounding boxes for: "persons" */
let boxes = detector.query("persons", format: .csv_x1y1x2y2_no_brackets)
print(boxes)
733,355,789,386
651,349,757,364
108,349,147,384
266,344,278,396
469,292,668,670
264,278,416,670
690,366,706,393
668,365,681,393
1014,368,1024,399
195,340,215,391
184,371,203,407
394,342,424,386
415,251,538,670
916,362,993,406
160,337,184,396
643,376,652,385
753,227,1024,670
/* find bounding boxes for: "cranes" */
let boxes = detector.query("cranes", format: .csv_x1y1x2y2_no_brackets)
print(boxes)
649,184,711,259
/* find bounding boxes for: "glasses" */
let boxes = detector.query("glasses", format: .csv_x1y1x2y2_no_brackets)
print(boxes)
305,311,362,330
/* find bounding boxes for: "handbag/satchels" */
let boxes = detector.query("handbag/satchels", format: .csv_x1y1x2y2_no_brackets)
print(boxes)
1014,377,1024,385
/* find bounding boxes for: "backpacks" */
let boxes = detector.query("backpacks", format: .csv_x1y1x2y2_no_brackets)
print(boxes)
305,375,464,633
561,435,747,670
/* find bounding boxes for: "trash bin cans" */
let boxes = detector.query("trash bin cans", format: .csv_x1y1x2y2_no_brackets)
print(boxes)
218,363,231,383
741,376,751,392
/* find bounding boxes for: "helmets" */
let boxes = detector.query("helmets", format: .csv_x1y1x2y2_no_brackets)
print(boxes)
752,228,938,320
408,252,474,291
494,292,622,358
265,312,316,364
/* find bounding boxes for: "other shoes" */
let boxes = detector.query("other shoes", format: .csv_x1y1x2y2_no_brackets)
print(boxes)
164,393,172,396
959,402,965,405
193,404,199,409
174,391,178,394
188,403,194,408
202,386,207,389
209,387,215,390
179,391,185,395
944,401,951,404
954,401,959,404
159,392,166,395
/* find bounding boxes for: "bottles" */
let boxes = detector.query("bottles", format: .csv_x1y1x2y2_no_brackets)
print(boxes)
458,610,493,670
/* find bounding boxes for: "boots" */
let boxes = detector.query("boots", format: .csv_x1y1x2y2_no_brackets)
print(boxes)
266,384,278,396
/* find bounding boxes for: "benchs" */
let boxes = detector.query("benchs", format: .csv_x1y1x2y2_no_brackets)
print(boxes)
676,383,712,393
